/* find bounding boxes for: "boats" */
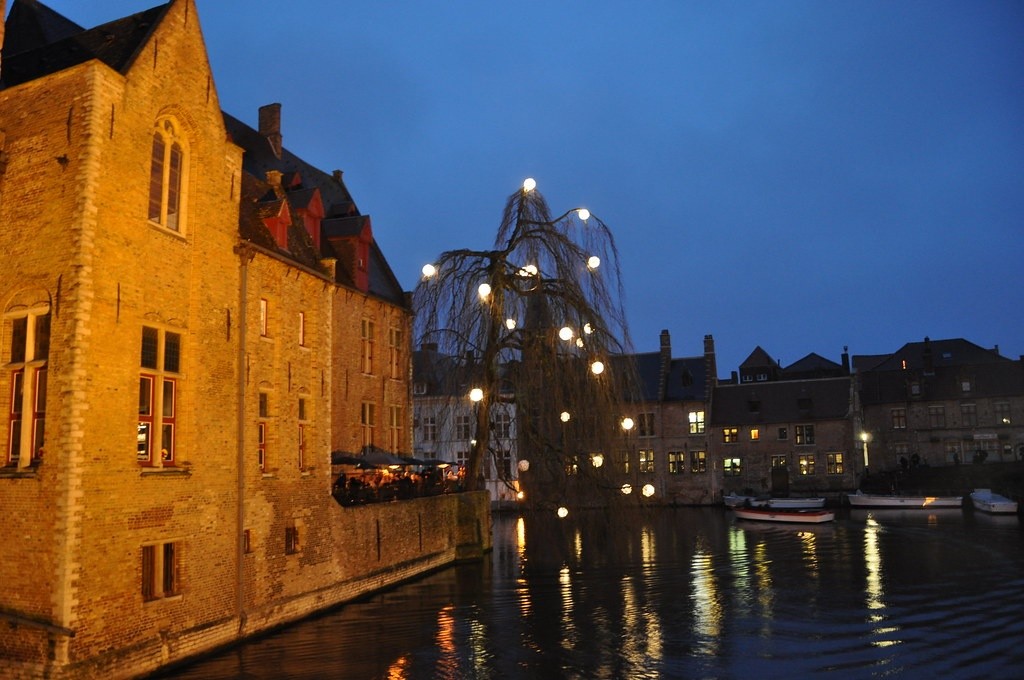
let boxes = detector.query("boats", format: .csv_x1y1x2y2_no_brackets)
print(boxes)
847,493,964,508
733,507,836,524
968,488,1017,513
722,490,826,510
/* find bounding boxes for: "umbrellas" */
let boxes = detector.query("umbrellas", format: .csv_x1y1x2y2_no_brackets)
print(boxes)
331,451,459,481
755,495,772,504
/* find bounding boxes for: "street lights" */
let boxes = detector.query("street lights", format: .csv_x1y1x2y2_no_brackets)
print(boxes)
861,432,871,479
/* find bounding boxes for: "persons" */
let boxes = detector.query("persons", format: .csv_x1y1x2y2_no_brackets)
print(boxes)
335,465,444,502
743,498,752,506
